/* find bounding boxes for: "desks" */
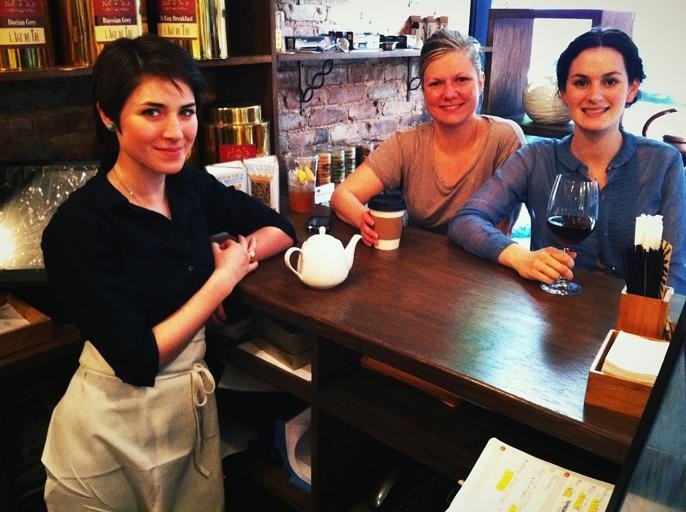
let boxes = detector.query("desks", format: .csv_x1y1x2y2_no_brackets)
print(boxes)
208,200,686,512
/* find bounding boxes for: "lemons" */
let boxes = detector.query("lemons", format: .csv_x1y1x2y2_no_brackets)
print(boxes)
295,168,314,182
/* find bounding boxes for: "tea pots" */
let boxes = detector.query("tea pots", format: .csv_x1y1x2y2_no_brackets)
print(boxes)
284,224,363,291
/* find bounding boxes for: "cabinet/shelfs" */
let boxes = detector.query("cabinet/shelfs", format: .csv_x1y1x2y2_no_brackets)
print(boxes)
0,0,286,512
478,8,686,171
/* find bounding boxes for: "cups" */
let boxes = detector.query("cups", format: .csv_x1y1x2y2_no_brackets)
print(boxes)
283,153,319,214
367,195,407,251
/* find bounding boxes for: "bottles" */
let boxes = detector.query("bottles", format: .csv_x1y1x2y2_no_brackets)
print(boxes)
315,147,355,184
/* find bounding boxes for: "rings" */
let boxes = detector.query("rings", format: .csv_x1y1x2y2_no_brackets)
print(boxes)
249,251,255,259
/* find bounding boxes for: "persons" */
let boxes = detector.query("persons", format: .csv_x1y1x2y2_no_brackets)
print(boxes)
447,27,686,295
330,29,527,250
41,36,298,512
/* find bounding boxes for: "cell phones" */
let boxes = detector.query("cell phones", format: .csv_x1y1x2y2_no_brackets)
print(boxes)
305,215,333,232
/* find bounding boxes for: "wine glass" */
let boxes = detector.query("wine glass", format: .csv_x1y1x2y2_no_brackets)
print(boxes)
538,175,600,297
